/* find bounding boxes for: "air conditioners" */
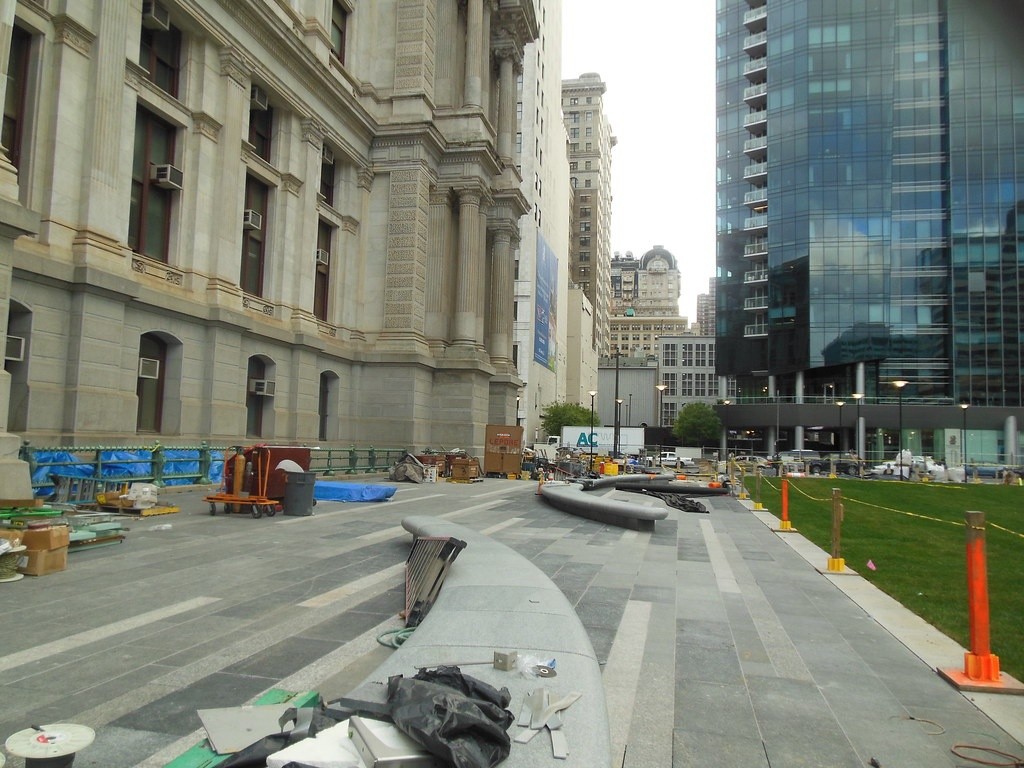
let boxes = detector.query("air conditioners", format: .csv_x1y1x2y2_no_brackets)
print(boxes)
251,87,268,110
139,357,159,379
250,379,275,396
244,209,262,230
317,249,329,265
5,335,25,361
322,146,333,164
142,0,170,31
151,164,184,190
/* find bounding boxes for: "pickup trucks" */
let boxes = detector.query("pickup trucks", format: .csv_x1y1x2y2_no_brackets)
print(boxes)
641,451,694,468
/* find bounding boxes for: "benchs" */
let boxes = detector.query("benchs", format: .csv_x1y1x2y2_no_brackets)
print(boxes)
292,515,612,768
540,473,675,532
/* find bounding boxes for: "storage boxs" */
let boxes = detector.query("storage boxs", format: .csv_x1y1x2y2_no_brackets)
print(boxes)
0,525,70,576
415,454,479,483
96,483,158,508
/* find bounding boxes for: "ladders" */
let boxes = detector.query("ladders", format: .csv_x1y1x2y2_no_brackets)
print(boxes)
554,467,574,481
404,536,468,630
42,472,129,506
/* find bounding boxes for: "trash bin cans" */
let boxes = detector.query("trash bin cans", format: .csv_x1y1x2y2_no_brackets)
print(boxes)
283,470,316,516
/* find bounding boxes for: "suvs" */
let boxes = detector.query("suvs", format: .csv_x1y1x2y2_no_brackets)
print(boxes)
809,452,860,475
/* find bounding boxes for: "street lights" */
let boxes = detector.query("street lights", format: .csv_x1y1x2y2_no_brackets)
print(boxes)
724,400,731,462
836,401,846,474
615,399,623,453
892,381,909,481
655,385,668,463
852,393,866,477
958,403,971,483
588,391,598,471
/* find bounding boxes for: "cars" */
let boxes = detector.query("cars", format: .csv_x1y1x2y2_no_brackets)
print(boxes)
911,456,937,467
966,462,1009,480
557,447,586,459
735,455,771,472
